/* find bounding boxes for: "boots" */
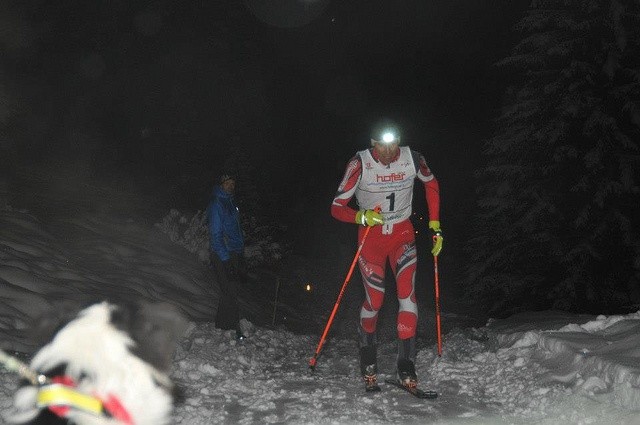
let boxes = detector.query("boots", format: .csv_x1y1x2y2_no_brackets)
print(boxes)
397,336,419,388
358,325,378,382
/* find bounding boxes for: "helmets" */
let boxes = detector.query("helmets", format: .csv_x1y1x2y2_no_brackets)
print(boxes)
371,124,401,146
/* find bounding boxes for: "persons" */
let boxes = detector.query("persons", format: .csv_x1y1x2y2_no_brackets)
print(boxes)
207,169,248,332
329,117,444,389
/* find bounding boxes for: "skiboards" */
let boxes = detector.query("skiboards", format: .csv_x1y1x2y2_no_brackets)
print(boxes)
366,378,437,399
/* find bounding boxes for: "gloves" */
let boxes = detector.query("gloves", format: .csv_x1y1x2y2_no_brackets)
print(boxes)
429,221,443,256
355,209,384,227
